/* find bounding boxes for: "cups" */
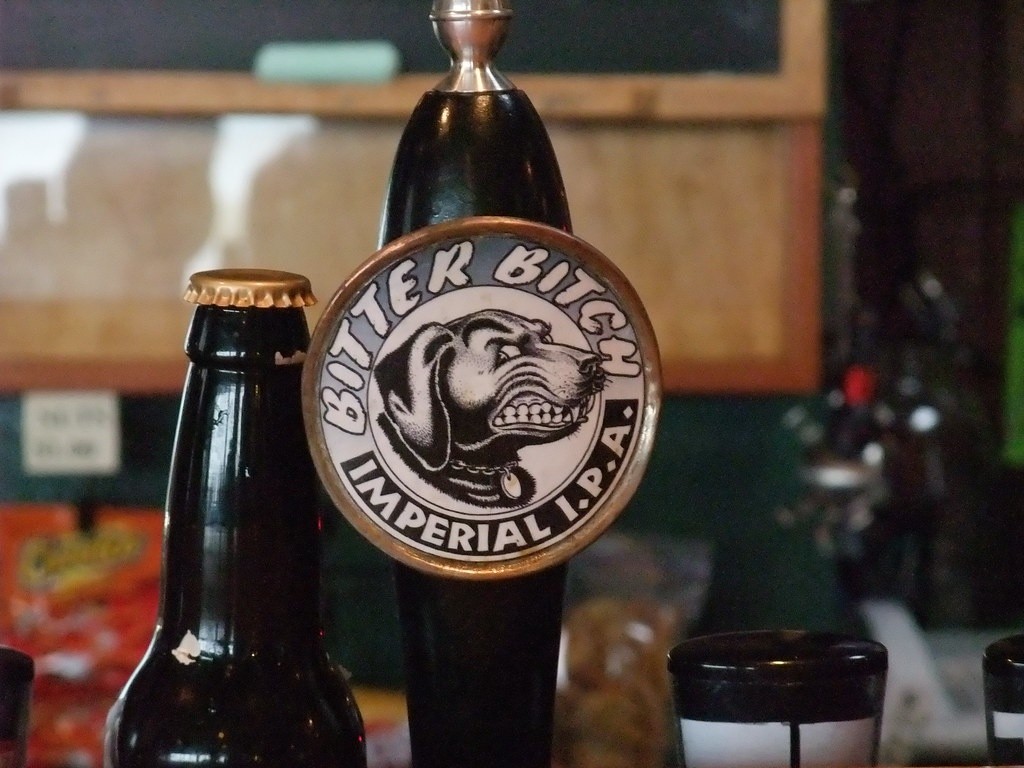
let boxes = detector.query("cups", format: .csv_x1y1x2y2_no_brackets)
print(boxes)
666,632,889,768
0,646,35,768
983,634,1024,768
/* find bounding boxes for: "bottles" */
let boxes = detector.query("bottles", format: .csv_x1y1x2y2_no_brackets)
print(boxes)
101,269,366,768
